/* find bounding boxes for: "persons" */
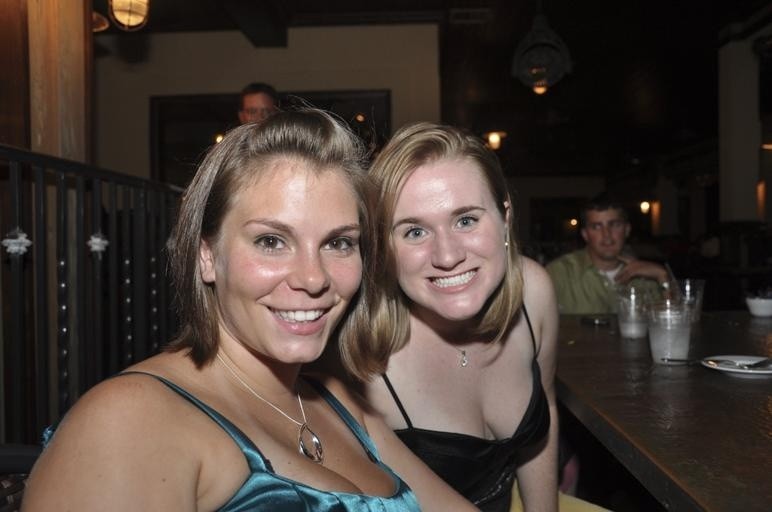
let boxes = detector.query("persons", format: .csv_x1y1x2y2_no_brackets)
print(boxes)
338,121,565,512
233,79,279,125
17,100,484,512
543,196,673,314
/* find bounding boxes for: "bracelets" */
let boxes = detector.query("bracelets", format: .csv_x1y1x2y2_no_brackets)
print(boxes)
658,279,672,291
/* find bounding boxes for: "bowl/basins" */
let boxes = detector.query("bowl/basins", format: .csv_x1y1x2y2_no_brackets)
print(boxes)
745,296,772,317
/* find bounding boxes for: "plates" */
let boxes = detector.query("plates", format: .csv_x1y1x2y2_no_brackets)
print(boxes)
700,354,771,379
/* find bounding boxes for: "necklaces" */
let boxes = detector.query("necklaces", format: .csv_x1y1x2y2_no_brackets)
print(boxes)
211,345,327,465
413,298,476,371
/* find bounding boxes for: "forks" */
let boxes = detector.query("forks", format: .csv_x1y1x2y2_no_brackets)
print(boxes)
660,357,770,370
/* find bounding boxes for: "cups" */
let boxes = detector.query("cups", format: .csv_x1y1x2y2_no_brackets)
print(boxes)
642,300,690,365
662,278,705,329
612,283,647,337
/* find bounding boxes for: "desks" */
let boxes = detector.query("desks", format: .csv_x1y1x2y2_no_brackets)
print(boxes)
555,314,772,511
